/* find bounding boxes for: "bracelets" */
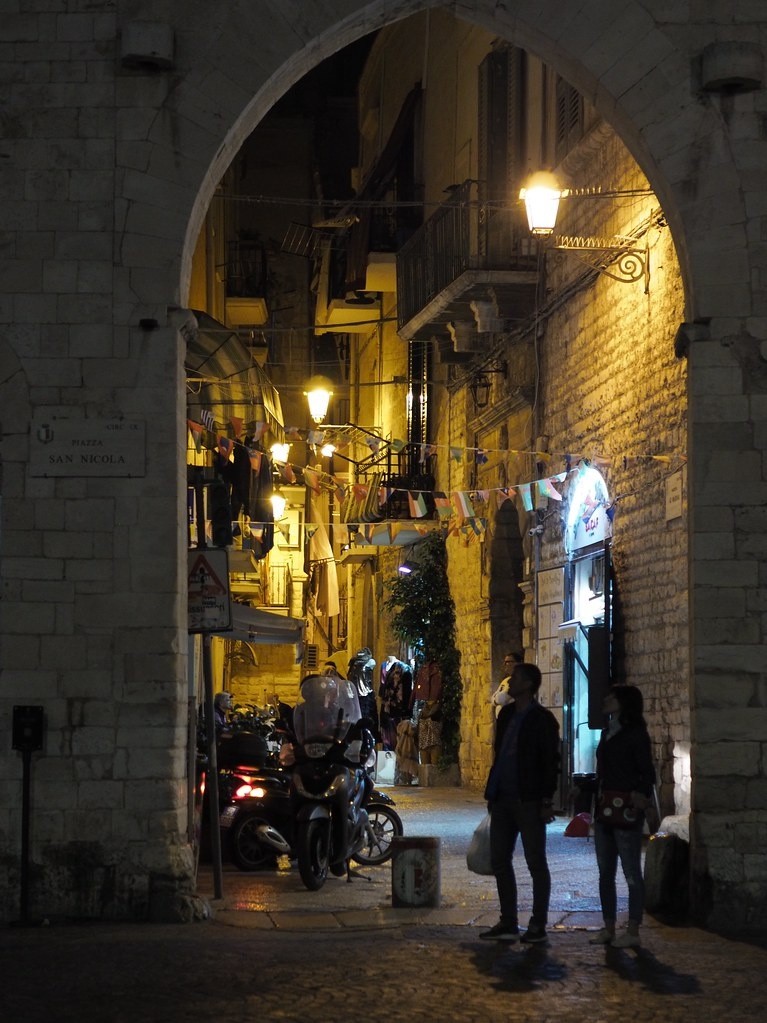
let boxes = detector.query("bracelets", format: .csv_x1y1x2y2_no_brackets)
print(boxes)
539,800,554,809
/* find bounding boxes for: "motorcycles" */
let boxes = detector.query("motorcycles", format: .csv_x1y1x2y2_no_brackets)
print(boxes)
199,693,405,891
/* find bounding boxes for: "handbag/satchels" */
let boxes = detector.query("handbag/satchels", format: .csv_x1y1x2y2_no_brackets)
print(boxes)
416,701,439,719
644,784,662,835
467,810,495,875
564,812,591,837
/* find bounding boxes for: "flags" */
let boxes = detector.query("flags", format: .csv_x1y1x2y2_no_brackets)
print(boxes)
187,410,612,544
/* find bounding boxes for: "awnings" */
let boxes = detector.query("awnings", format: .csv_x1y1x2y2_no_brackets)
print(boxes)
185,309,286,430
210,603,308,667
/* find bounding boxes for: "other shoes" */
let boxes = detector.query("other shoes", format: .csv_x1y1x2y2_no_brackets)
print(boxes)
330,855,345,876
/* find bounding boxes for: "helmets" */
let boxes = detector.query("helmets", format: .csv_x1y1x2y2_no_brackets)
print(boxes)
335,729,375,766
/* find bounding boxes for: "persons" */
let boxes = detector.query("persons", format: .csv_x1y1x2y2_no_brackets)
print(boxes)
347,647,443,764
284,662,346,769
588,684,654,946
478,653,560,942
214,692,231,727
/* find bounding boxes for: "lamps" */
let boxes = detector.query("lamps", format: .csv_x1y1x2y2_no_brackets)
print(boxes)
469,360,509,408
272,480,287,521
518,168,654,236
398,542,420,574
301,375,446,430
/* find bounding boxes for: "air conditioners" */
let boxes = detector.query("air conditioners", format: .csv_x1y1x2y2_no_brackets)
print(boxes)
300,643,319,671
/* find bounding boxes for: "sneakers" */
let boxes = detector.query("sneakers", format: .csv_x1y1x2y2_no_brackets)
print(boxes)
520,923,549,941
589,930,616,944
479,920,520,939
610,932,641,947
